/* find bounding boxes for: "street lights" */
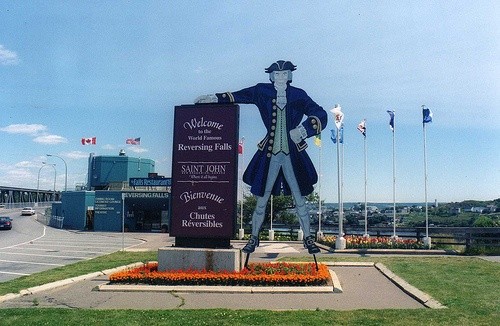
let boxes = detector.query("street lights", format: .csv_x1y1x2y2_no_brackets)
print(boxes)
35,163,56,207
42,161,57,201
46,154,68,191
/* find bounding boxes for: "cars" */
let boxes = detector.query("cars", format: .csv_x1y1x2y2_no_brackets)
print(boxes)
0,217,13,230
21,207,36,215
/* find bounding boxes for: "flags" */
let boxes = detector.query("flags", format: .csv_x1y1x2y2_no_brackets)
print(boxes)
331,128,343,143
423,108,432,123
315,134,321,146
126,137,140,144
82,137,96,145
239,141,242,153
357,121,366,136
389,113,394,131
